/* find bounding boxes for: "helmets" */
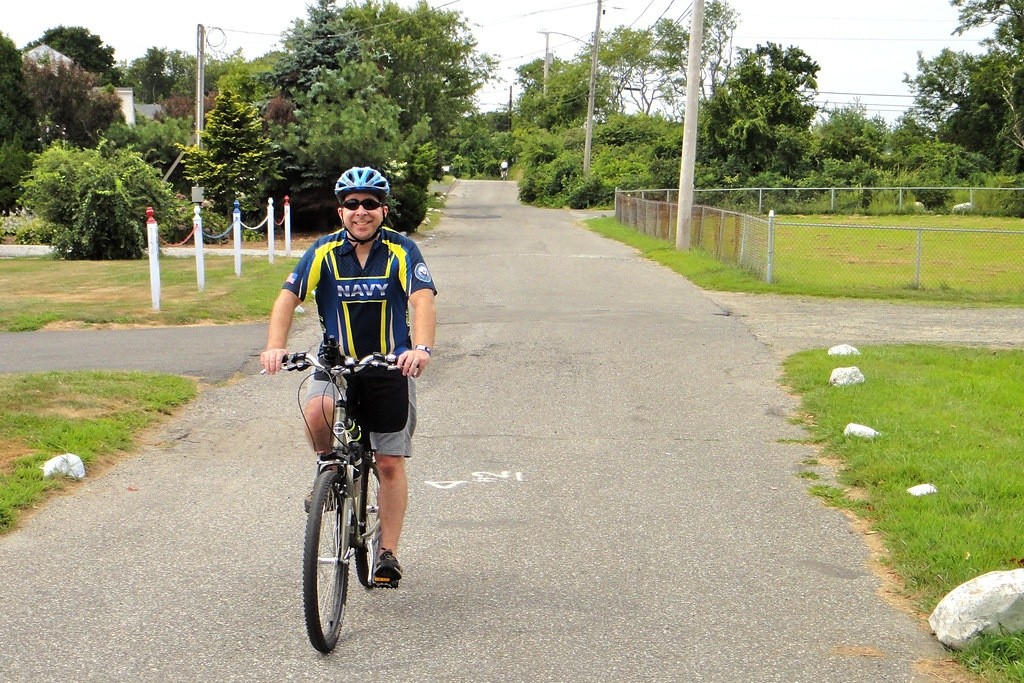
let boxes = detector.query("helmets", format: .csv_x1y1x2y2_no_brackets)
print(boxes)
335,166,390,197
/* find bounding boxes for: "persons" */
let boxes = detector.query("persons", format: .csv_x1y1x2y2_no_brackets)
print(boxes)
260,167,438,579
500,159,508,180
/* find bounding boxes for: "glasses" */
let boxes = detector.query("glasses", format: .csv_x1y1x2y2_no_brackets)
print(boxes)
340,198,381,211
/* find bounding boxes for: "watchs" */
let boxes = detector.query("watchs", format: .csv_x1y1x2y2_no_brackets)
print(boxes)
412,344,432,358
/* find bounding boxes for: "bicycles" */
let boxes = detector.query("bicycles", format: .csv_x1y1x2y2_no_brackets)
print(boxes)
500,168,506,180
260,351,419,654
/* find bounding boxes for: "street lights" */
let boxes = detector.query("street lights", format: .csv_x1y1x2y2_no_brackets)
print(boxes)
539,29,598,175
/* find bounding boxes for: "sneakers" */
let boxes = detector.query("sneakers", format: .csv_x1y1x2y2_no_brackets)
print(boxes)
304,487,333,513
373,547,403,581
